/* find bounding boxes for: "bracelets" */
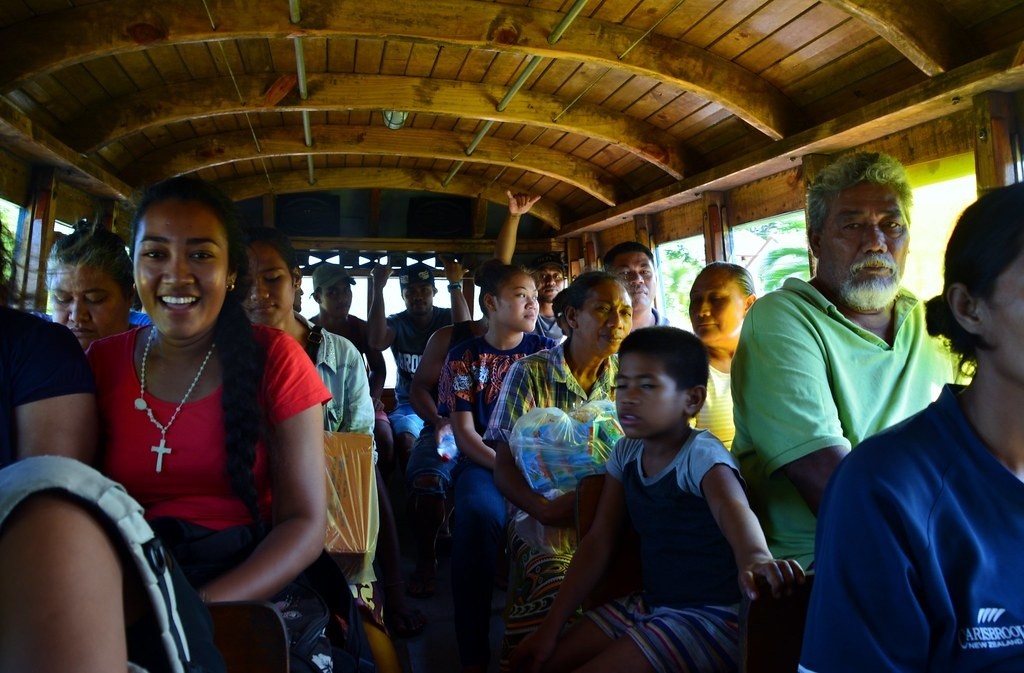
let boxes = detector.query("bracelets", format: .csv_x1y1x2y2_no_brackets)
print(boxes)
448,283,461,290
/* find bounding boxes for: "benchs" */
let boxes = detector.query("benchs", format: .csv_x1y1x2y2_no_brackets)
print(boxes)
325,431,815,673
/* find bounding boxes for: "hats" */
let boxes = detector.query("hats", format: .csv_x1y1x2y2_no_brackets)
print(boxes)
313,262,356,292
399,262,438,300
531,253,564,272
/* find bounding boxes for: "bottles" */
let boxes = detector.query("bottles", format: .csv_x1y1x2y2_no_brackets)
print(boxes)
436,426,459,462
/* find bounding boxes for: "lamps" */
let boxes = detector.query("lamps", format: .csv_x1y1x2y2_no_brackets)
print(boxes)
380,110,409,130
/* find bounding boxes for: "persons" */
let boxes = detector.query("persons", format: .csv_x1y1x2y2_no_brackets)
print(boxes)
406,315,491,599
45,220,139,351
481,272,635,673
0,454,221,673
0,227,98,471
728,152,953,573
308,262,404,490
688,261,757,452
797,182,1024,673
601,240,672,338
367,253,471,538
507,326,804,673
80,176,376,673
499,188,569,344
290,268,429,639
235,228,379,475
436,258,556,673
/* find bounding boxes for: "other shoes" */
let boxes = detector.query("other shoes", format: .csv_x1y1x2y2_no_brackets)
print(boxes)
406,559,441,599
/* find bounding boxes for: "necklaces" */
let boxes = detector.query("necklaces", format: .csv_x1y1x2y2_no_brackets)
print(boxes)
139,323,219,475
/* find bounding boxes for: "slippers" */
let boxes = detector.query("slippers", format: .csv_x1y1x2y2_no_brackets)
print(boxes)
386,608,424,639
